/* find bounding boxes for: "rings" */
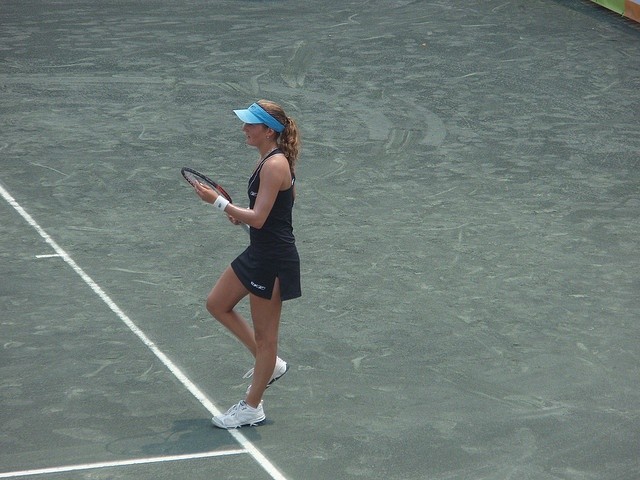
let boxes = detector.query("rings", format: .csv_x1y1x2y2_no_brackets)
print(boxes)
196,189,199,193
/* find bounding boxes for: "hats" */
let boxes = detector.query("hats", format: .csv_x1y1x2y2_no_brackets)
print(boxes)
232,102,286,133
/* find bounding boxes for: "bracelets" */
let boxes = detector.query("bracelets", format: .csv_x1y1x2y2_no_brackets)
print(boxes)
212,195,230,211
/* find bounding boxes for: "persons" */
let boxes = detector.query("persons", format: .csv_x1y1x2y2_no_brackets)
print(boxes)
193,99,302,429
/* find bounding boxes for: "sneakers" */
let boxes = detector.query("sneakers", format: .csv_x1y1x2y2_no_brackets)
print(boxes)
242,355,290,395
210,399,266,430
